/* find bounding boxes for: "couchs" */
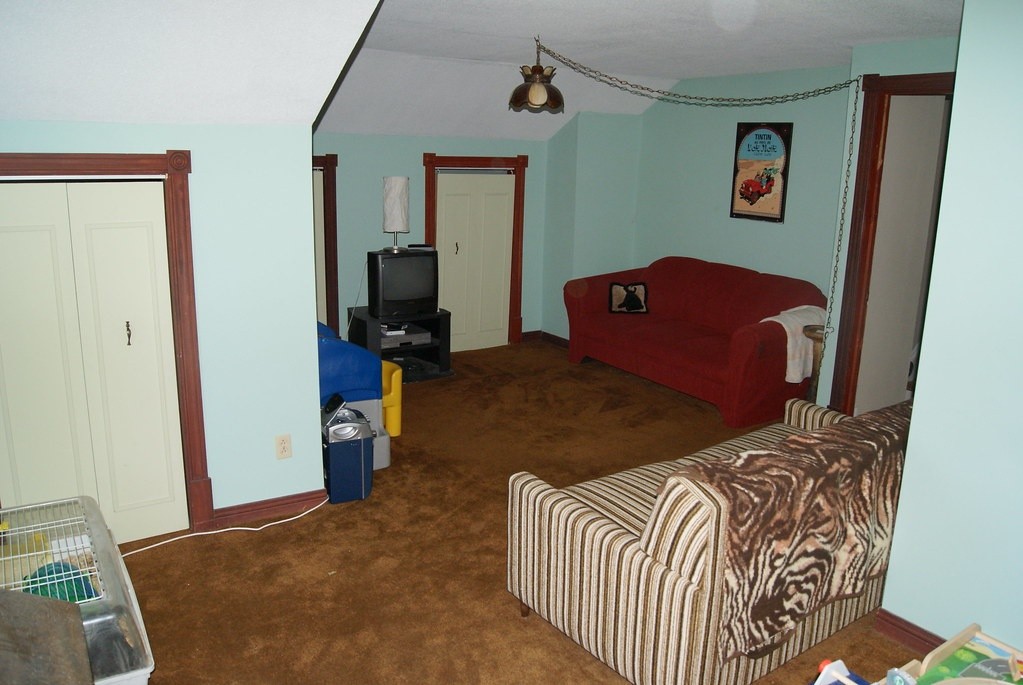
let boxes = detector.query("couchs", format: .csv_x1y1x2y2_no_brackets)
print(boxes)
506,399,916,685
562,256,828,428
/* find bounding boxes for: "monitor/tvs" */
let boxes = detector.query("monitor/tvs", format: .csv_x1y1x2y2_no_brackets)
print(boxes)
366,250,439,320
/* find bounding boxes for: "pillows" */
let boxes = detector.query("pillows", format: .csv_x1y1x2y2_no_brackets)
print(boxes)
608,281,650,313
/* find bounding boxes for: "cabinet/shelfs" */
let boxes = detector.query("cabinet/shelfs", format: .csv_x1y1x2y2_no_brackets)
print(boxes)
347,304,455,389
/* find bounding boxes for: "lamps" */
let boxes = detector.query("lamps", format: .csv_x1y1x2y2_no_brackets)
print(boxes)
382,175,411,255
508,38,566,116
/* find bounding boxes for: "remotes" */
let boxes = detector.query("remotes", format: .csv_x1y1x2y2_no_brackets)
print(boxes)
407,244,432,248
